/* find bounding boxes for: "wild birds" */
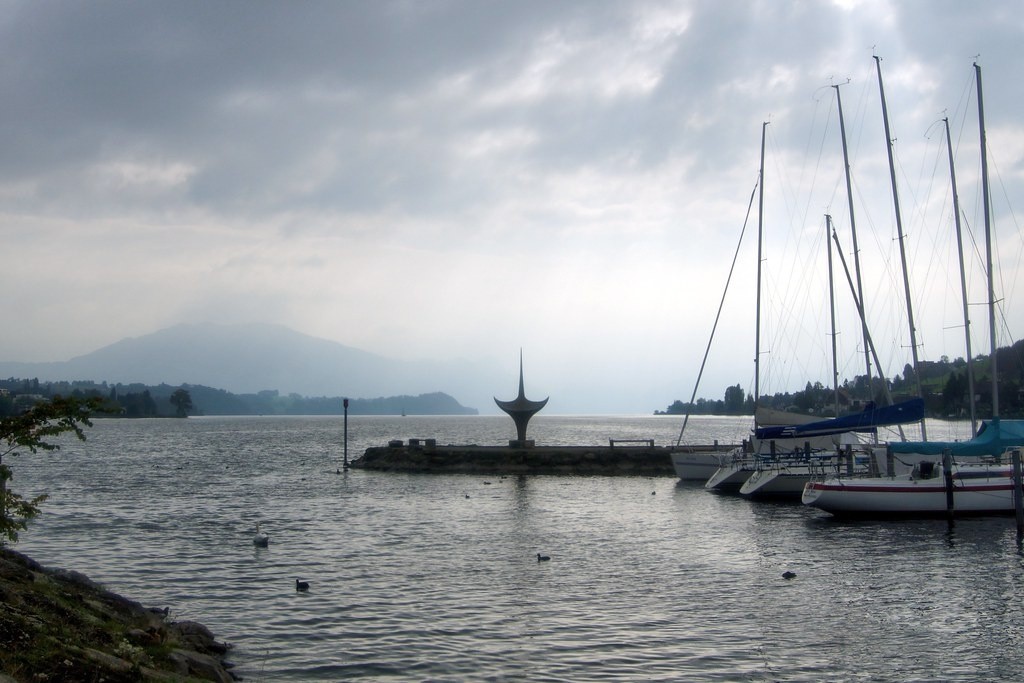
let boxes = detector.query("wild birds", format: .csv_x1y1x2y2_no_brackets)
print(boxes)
536,553,551,561
781,571,797,579
296,578,308,588
252,524,270,545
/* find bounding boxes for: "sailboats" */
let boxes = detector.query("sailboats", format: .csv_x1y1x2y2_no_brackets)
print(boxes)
668,56,1024,519
